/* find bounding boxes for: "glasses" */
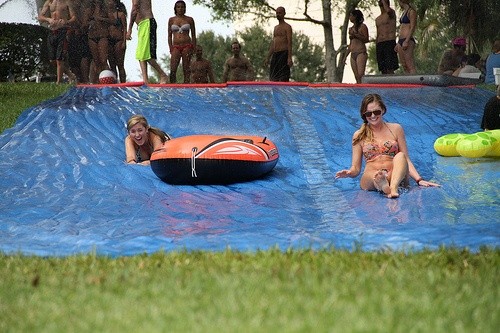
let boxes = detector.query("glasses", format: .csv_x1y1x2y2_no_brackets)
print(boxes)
365,110,381,117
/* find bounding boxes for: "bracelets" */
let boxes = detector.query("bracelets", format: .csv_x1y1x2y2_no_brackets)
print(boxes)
415,177,424,185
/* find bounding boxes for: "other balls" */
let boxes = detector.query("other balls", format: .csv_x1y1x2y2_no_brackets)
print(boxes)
99,69,116,84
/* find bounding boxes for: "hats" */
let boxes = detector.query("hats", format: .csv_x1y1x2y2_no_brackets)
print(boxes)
453,37,466,45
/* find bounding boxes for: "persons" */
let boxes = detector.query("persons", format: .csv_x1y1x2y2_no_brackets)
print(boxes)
438,37,500,84
168,1,196,83
126,0,168,84
37,0,128,84
222,42,255,82
335,93,440,197
189,45,215,83
125,114,170,165
375,0,399,75
394,0,417,75
264,7,293,82
342,10,369,84
480,85,500,131
